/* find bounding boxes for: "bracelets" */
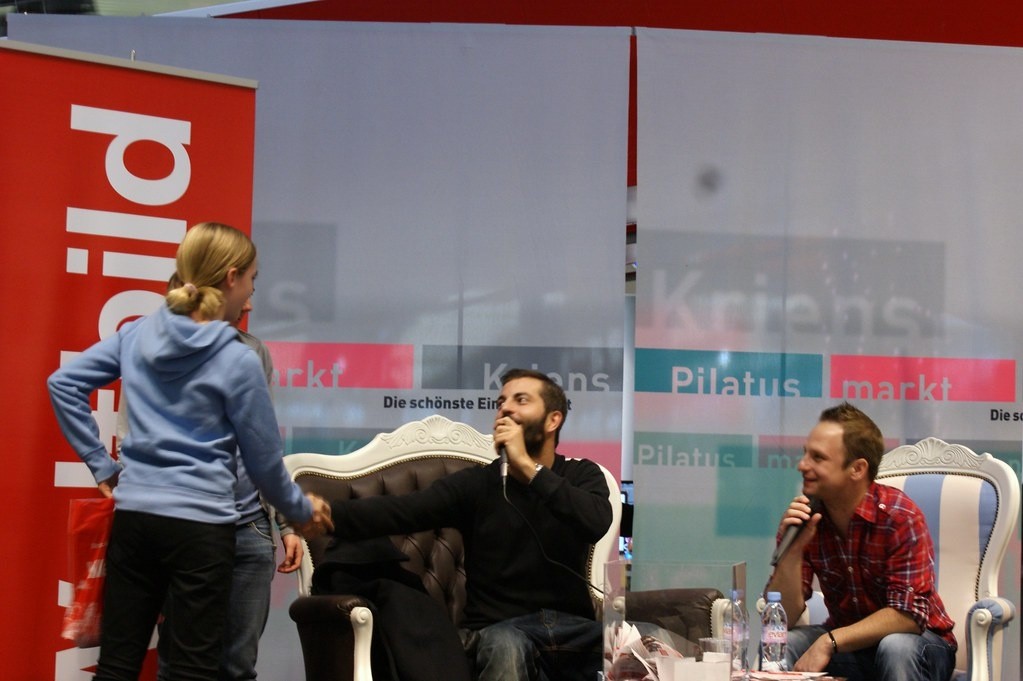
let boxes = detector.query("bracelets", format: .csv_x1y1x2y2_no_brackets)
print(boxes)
529,465,542,483
828,632,838,653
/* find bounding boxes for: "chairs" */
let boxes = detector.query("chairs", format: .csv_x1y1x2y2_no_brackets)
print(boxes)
260,414,620,681
813,437,1021,681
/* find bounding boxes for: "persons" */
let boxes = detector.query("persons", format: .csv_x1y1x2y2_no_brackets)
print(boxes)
169,273,304,681
47,222,334,681
764,403,958,681
304,369,612,681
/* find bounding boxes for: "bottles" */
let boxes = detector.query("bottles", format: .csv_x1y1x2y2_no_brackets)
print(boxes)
762,592,788,674
723,590,751,677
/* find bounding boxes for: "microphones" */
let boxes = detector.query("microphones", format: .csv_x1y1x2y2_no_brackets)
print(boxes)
499,443,509,485
770,495,819,568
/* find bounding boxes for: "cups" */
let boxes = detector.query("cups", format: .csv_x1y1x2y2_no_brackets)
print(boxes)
698,636,731,661
